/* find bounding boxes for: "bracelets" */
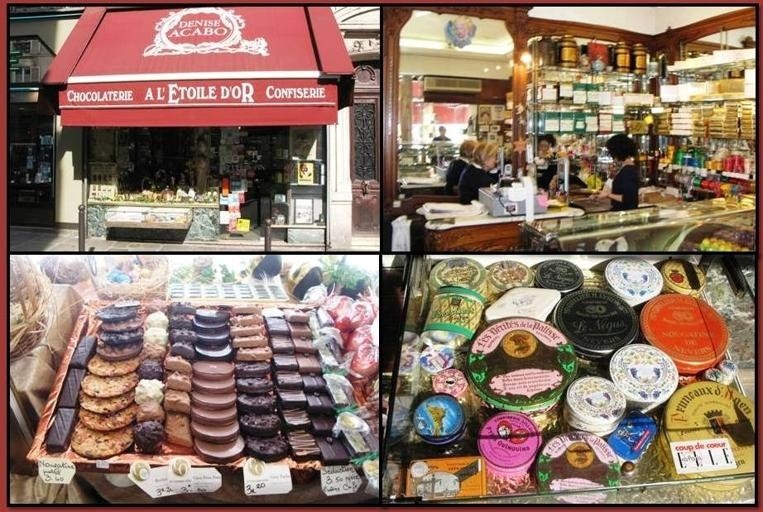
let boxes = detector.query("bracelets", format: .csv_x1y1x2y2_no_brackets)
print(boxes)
606,194,611,198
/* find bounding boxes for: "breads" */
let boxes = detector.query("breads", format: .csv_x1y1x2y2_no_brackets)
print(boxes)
47,302,370,466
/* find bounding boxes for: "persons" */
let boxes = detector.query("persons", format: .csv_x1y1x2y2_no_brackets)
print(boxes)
430,126,453,166
529,134,561,190
590,134,639,214
444,139,477,195
455,142,501,206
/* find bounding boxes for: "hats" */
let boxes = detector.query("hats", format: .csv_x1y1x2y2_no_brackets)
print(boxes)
458,139,480,160
473,140,498,165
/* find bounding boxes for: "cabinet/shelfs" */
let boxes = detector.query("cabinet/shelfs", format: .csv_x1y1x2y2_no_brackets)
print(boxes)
518,67,756,145
